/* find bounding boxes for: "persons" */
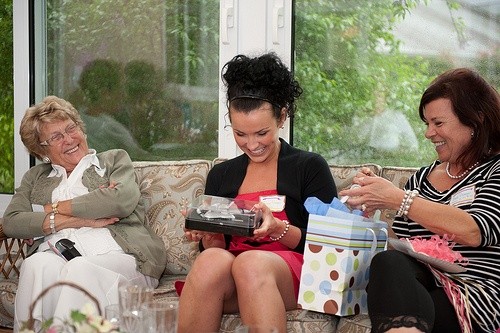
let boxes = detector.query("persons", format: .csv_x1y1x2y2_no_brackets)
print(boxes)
2,94,167,333
340,69,500,333
352,81,417,158
74,59,166,164
176,51,338,333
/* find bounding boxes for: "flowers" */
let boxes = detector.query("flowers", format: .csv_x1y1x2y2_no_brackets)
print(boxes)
17,309,118,333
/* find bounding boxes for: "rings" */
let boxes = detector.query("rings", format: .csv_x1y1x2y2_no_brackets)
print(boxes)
362,204,366,212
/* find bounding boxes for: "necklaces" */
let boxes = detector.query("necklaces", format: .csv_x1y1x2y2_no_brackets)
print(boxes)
446,148,493,178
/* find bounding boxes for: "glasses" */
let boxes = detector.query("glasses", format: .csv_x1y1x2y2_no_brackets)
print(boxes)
39,122,79,146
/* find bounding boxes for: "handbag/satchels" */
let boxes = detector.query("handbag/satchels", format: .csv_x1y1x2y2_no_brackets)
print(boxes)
297,195,389,316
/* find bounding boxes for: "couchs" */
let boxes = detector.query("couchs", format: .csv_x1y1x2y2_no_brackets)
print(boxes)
0,158,425,333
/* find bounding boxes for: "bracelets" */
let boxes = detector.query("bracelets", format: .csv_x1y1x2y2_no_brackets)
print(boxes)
52,200,58,214
270,220,290,241
398,189,419,216
50,212,55,233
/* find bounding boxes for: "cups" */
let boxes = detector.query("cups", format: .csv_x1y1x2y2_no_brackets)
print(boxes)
103,300,179,333
